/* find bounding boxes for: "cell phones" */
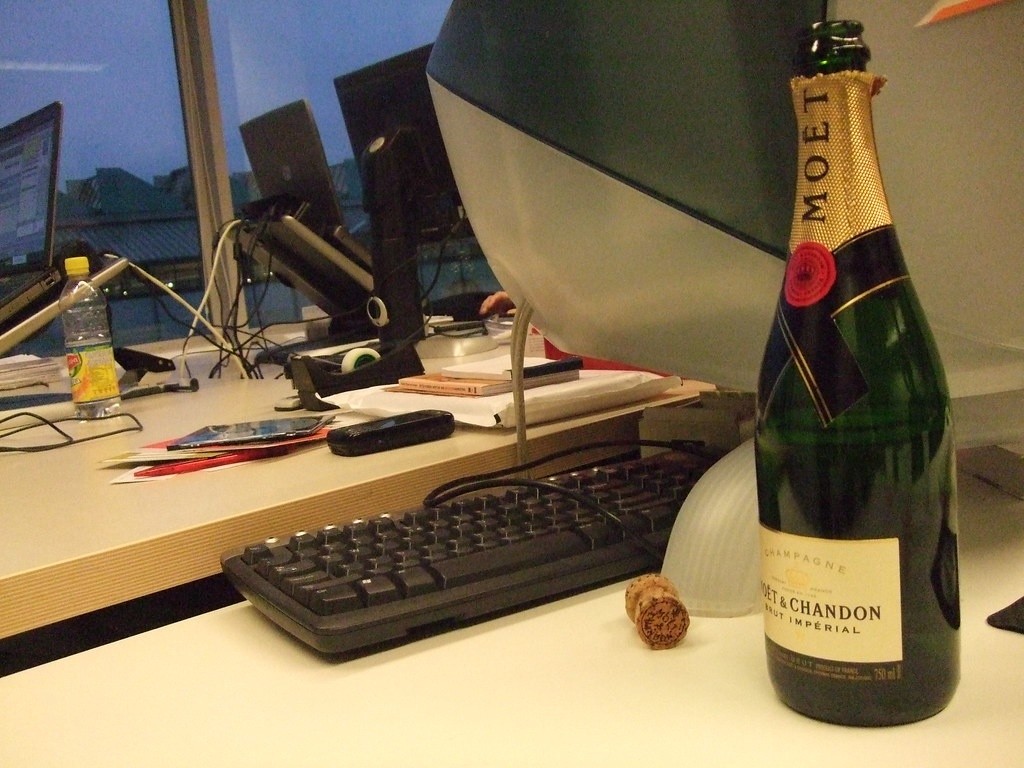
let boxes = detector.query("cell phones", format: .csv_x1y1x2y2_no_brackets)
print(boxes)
167,417,329,452
326,410,455,457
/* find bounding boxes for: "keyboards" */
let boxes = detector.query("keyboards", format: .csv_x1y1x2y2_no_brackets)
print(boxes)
221,448,729,654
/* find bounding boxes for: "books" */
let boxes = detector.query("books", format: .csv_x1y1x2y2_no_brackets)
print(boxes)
397,353,582,399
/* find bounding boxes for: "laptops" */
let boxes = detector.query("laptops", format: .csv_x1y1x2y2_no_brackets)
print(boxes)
239,101,372,271
0,100,64,322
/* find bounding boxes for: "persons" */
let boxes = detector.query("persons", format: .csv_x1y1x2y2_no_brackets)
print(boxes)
479,291,517,315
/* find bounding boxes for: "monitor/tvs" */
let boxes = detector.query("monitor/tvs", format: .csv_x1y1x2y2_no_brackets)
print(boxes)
334,42,499,360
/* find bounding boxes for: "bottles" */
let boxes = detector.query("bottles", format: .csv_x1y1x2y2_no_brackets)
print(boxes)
59,257,121,420
752,19,961,728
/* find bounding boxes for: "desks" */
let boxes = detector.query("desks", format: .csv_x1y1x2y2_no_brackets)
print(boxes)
0,443,1024,768
0,334,715,639
